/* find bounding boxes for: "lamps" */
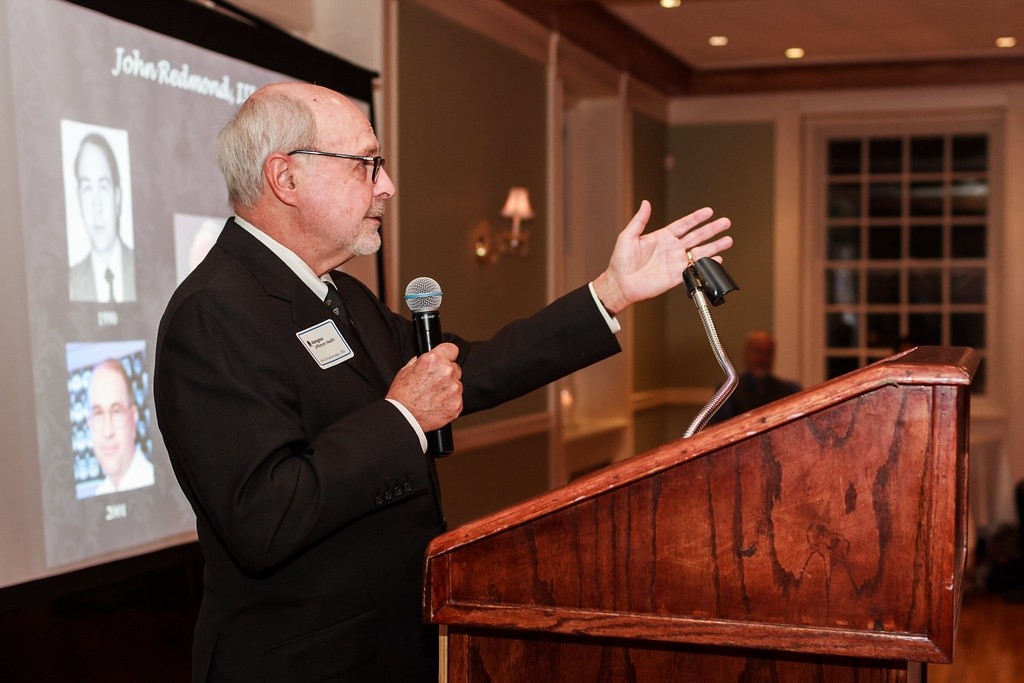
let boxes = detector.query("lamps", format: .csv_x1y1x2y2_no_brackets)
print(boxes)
474,187,535,267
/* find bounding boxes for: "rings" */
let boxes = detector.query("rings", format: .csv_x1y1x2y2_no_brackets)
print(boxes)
687,251,692,263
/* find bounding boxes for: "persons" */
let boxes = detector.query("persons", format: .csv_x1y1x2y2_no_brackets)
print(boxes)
711,331,801,423
152,84,733,683
67,133,137,302
88,359,155,495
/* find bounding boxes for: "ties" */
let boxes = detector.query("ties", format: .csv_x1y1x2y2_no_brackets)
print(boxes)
104,268,119,303
324,281,360,344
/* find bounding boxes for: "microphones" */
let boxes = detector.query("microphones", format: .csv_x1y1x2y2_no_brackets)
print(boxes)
404,277,454,459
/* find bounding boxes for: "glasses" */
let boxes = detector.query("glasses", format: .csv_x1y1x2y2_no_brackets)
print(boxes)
288,149,386,184
